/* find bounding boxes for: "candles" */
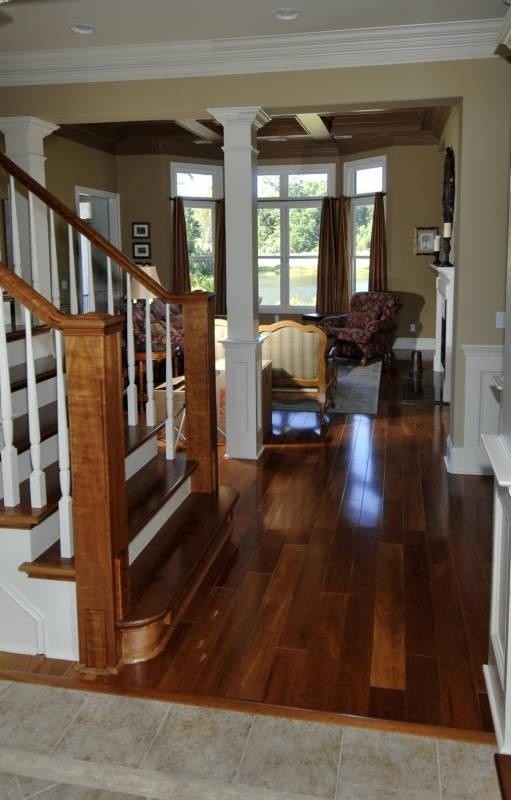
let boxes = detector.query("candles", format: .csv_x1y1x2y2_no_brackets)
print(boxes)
433,235,442,252
444,222,453,238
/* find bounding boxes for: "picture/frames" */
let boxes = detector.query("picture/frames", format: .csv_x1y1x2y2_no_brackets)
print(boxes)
414,226,439,256
132,222,152,260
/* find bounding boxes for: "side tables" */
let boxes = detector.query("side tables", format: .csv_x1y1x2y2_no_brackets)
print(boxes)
127,342,182,414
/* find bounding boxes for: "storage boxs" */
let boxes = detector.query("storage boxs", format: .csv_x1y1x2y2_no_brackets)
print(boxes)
152,357,274,444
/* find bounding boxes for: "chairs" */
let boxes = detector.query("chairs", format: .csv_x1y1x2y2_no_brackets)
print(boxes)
259,318,340,428
317,291,404,367
126,295,184,359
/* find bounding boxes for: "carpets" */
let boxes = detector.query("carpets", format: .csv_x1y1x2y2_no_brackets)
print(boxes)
271,356,384,416
